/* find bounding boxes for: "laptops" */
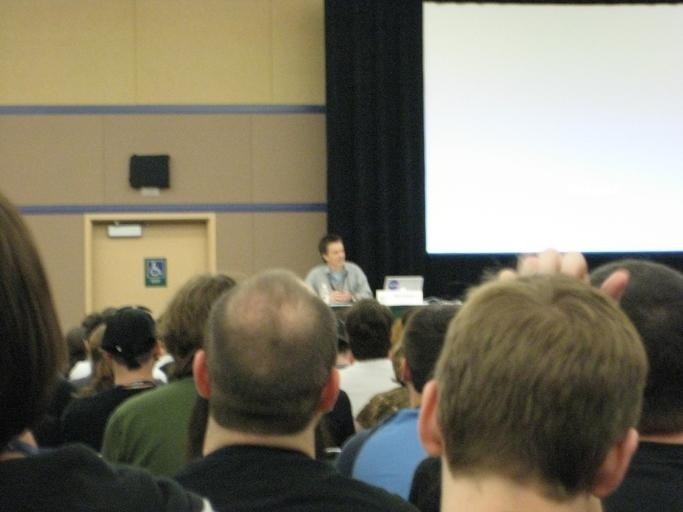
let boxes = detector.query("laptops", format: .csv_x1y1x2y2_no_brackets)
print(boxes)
383,275,424,291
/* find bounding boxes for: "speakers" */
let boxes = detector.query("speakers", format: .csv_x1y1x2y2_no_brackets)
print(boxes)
128,153,170,189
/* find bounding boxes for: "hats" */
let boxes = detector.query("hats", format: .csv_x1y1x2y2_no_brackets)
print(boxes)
98,305,157,369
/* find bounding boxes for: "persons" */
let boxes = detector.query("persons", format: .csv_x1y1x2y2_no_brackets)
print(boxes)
179,271,419,512
0,192,212,511
334,298,463,498
58,273,324,475
306,233,371,304
410,251,682,511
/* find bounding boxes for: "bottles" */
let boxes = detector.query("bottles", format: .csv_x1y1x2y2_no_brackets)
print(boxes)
320,284,330,305
